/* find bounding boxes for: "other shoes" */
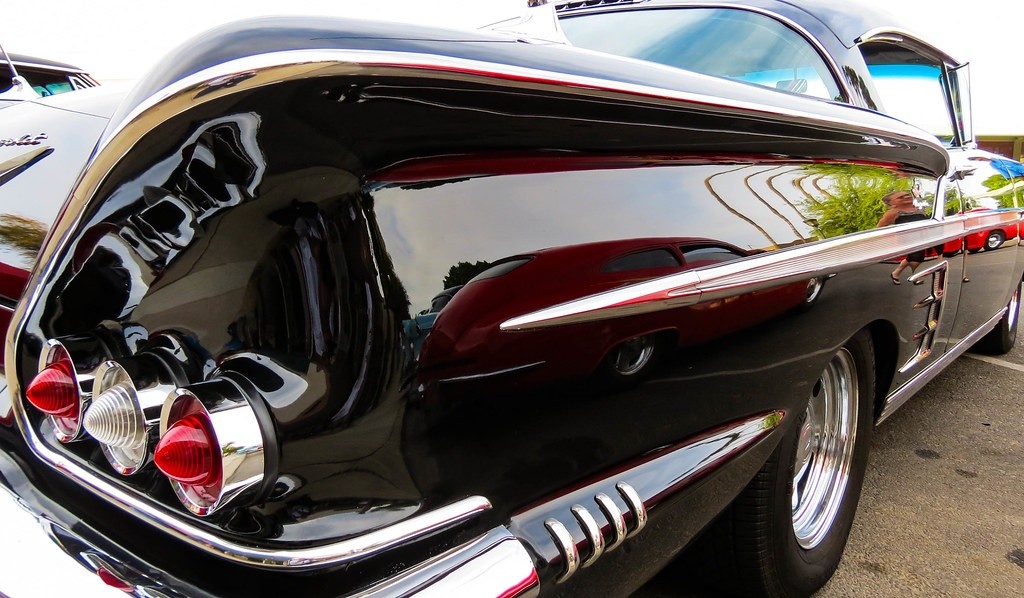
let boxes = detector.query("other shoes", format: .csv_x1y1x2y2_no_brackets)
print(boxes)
963,277,970,282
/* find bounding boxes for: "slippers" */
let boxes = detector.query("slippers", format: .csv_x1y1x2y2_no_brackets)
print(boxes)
891,273,901,284
914,280,923,285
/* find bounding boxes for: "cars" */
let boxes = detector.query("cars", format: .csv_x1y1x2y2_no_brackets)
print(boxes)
414,238,825,394
400,312,438,334
884,206,1024,263
0,0,1024,598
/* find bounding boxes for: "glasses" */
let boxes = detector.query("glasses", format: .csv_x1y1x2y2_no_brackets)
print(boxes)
897,194,910,199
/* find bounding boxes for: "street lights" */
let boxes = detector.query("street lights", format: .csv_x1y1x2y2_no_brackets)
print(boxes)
804,220,825,238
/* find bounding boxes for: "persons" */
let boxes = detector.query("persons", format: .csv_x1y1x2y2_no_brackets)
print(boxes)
874,189,933,285
